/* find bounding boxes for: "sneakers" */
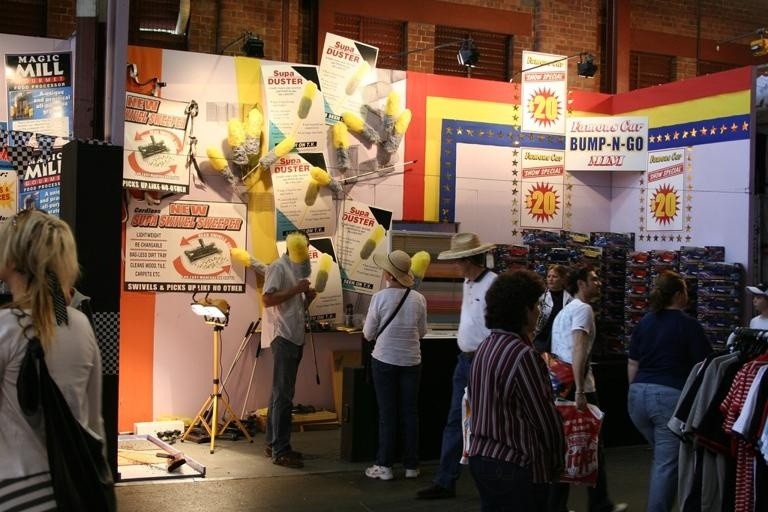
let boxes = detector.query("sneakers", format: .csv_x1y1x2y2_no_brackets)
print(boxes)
264,444,304,468
405,468,420,479
414,481,457,499
364,465,394,480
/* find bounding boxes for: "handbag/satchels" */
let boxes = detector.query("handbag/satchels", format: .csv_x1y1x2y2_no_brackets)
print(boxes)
540,351,575,401
17,336,118,512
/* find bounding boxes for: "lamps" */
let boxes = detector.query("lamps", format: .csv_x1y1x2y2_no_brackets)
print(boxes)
182,287,254,453
390,35,479,68
512,50,597,79
222,33,266,58
717,28,768,56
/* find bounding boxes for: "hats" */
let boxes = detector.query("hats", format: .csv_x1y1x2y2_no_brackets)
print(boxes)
372,249,416,288
745,283,768,297
437,232,498,261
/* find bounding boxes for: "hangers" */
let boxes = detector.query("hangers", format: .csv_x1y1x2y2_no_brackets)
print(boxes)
730,327,768,355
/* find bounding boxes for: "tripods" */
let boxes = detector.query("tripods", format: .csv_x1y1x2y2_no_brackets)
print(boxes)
182,327,253,454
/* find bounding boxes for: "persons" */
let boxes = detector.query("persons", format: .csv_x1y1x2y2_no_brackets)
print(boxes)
531,263,572,354
748,281,767,338
362,249,427,480
260,232,317,466
626,269,710,511
413,232,500,500
0,209,114,510
466,270,569,512
552,266,628,512
